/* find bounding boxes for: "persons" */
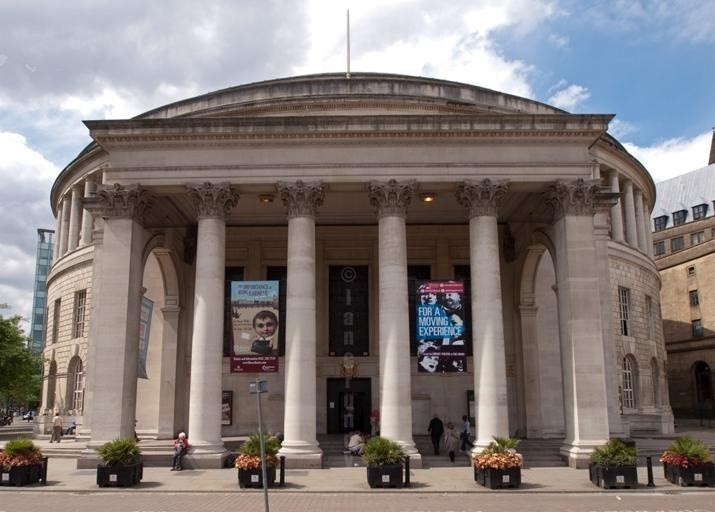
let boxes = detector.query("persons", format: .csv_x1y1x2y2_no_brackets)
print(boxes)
64,421,76,435
50,413,62,443
171,431,189,471
458,415,473,452
134,431,140,442
444,424,459,462
347,430,364,456
416,281,467,372
427,413,444,455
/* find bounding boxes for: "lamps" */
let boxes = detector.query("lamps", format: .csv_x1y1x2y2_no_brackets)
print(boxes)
257,194,275,204
419,192,440,204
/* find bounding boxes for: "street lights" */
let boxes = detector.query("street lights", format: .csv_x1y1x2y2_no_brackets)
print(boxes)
249,377,271,511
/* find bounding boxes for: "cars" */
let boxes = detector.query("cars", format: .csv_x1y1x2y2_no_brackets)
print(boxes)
24,411,36,420
